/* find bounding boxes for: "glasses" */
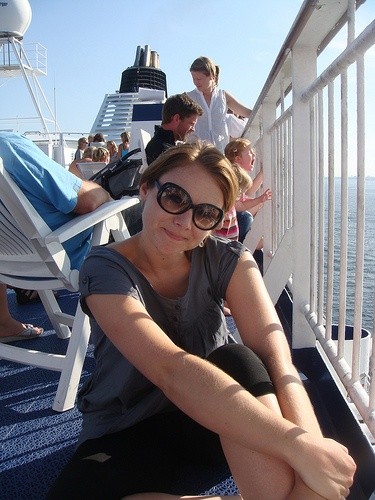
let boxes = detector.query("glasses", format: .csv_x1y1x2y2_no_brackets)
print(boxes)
153,177,224,231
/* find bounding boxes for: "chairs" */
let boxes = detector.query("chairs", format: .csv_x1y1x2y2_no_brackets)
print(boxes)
0,156,140,412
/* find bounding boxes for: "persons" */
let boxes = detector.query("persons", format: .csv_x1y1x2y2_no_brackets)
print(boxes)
106,140,118,163
144,93,254,244
92,147,110,164
224,108,249,142
0,283,45,344
116,131,131,159
75,137,89,160
69,146,96,180
87,134,95,147
210,137,273,316
47,138,358,500
182,55,254,158
0,129,116,285
90,133,107,148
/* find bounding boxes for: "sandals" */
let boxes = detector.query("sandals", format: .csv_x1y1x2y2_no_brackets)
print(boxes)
16,290,60,306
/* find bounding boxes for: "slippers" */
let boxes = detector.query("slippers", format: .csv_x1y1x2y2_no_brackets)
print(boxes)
0,324,44,343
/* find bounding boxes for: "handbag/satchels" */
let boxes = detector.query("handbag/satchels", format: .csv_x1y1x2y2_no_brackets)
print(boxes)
88,147,145,200
